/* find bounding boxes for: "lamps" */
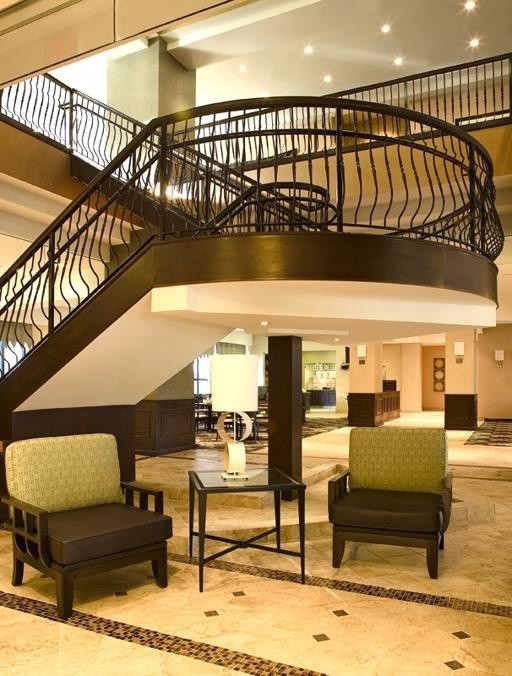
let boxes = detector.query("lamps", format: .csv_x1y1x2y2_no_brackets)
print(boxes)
210,354,259,482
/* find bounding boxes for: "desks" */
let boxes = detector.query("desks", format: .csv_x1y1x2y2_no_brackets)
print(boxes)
188,469,307,593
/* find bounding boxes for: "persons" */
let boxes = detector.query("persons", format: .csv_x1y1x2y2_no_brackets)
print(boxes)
205,393,212,403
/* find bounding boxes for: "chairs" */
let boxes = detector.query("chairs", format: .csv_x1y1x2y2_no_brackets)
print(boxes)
195,394,259,442
327,426,453,579
0,432,172,620
